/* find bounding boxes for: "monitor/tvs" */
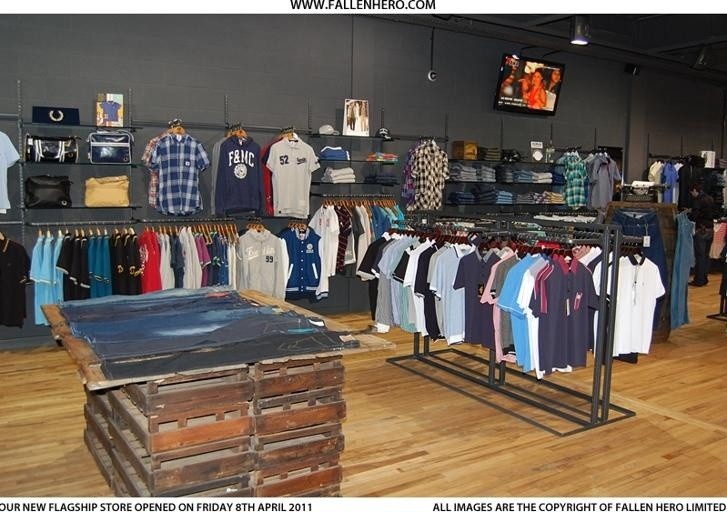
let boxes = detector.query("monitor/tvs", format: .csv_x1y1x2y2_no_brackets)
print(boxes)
494,52,566,117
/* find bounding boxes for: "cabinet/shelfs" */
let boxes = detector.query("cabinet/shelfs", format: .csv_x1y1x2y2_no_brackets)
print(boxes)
20,121,144,220
310,131,398,186
441,154,568,207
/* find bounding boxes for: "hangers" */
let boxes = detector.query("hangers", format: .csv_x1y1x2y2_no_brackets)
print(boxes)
33,212,265,244
384,211,644,260
286,217,307,233
227,120,246,140
320,193,396,211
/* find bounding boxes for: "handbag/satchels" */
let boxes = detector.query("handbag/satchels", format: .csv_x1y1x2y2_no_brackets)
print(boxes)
25,133,78,164
24,175,72,208
83,175,130,207
86,128,134,164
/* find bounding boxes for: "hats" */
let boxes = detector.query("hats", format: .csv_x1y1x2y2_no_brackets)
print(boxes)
319,124,339,135
375,127,392,140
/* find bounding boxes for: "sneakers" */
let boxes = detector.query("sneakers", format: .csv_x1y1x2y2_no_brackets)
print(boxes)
688,278,708,287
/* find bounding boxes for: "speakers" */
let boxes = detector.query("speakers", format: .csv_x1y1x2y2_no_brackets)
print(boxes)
624,63,642,75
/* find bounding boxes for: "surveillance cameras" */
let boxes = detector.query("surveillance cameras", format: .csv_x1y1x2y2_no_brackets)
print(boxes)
428,71,438,82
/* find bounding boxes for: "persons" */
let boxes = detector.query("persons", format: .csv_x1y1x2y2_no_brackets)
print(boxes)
519,67,547,110
683,183,715,288
545,69,561,91
347,101,367,132
